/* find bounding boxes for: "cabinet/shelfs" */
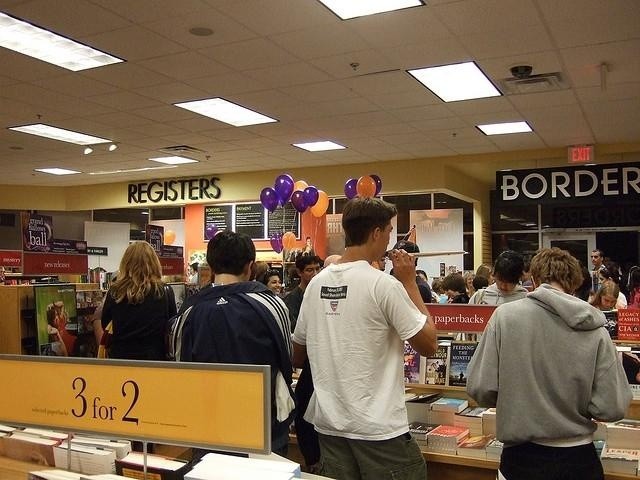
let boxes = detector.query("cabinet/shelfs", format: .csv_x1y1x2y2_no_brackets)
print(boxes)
35,281,106,357
8,272,83,284
283,368,639,479
0,440,92,479
1,282,100,356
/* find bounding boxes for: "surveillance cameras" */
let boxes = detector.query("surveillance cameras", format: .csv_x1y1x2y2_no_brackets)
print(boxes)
508,63,534,79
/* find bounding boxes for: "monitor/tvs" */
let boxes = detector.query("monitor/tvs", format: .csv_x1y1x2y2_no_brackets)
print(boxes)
166,282,186,317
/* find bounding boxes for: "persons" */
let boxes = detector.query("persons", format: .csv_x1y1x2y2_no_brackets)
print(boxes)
290,196,439,480
91,240,178,362
169,231,293,467
431,273,470,304
466,247,633,480
282,256,321,334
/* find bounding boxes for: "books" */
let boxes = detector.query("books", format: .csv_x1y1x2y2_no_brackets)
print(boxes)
403,333,504,462
115,451,300,480
0,425,91,480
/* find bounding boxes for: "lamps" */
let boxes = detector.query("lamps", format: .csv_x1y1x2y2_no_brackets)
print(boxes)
82,141,121,156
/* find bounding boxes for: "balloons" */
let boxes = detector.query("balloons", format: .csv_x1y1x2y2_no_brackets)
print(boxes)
206,173,383,254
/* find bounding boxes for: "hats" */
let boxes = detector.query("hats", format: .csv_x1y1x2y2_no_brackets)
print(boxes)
386,240,421,261
